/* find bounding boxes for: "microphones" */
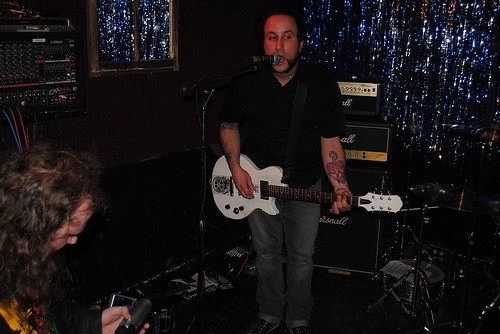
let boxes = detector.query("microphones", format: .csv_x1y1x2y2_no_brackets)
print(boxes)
243,54,280,66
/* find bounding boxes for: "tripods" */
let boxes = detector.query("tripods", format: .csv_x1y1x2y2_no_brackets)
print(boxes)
354,199,497,334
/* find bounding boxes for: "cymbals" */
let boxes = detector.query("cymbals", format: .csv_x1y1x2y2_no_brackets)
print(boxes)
429,197,482,214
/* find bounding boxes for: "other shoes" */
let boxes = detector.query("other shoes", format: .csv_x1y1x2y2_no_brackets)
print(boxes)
243,318,280,334
281,319,310,334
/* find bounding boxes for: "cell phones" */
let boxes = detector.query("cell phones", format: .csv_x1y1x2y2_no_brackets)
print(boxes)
114,300,153,334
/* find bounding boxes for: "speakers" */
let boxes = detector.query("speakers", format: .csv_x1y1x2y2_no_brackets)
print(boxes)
312,168,388,274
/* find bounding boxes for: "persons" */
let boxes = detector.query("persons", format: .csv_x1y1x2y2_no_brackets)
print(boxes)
0,142,150,334
218,4,353,334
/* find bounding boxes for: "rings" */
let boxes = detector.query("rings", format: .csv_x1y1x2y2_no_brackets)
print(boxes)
345,205,351,211
339,208,345,213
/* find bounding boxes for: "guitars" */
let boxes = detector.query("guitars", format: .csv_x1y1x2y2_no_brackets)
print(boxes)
212,153,404,221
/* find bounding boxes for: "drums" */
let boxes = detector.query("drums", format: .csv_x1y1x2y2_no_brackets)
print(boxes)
385,258,446,320
475,126,500,156
